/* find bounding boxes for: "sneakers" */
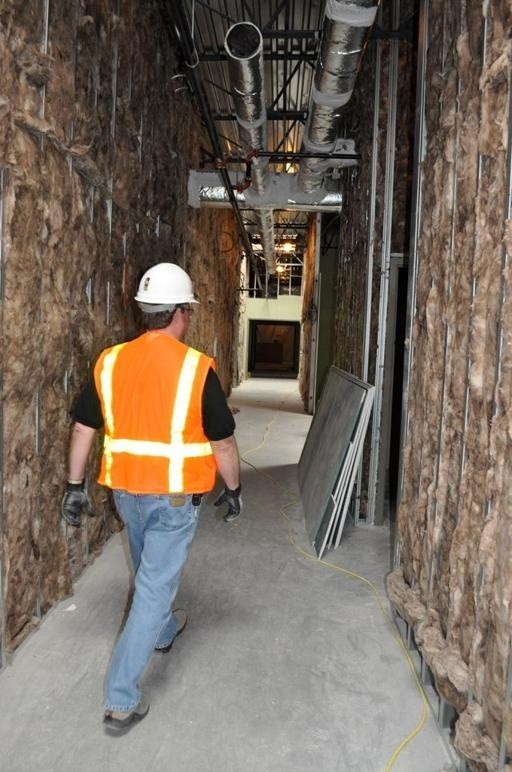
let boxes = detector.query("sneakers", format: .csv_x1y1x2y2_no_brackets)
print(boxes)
103,700,149,730
153,608,187,654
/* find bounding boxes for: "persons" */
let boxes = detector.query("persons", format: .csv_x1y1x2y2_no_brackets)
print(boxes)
61,261,241,731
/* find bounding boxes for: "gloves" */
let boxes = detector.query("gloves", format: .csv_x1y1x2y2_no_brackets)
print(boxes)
61,477,95,527
214,486,244,522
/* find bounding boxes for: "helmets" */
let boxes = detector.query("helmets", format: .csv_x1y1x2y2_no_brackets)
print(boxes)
134,262,199,310
